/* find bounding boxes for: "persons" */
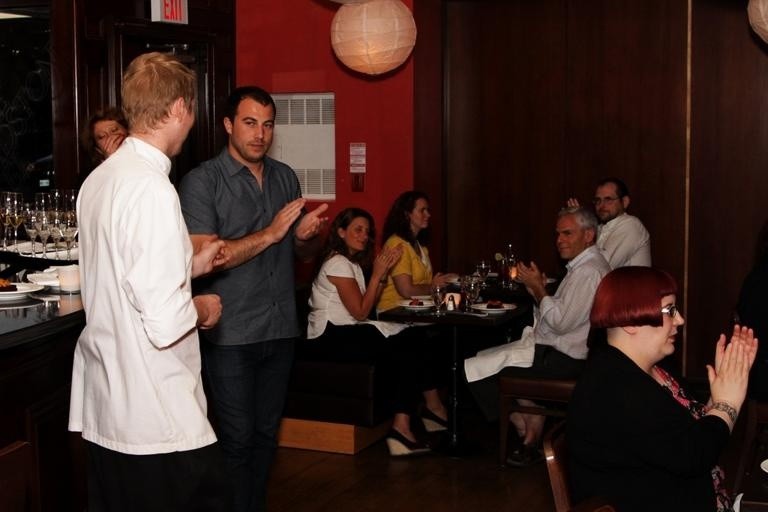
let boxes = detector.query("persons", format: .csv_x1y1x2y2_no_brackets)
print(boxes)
568,180,652,269
76,104,129,161
461,205,612,447
67,52,231,512
176,83,330,509
555,265,760,512
304,207,450,457
373,191,459,322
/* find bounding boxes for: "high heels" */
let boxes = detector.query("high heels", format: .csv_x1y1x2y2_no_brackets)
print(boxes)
386,428,430,455
421,406,449,433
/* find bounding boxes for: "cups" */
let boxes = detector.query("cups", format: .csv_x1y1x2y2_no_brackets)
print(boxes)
60,267,81,294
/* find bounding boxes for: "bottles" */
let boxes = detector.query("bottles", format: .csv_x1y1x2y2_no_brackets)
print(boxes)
447,295,455,311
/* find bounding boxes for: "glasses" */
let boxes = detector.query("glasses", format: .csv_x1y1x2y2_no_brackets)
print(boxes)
594,196,618,205
662,304,677,319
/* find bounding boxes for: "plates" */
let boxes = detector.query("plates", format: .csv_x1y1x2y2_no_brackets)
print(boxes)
397,299,435,311
447,278,461,286
1,272,61,302
515,278,555,285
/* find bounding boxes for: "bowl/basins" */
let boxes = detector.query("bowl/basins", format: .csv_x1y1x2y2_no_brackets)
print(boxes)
444,292,461,308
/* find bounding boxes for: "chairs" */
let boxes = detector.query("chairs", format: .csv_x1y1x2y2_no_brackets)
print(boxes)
272,239,767,510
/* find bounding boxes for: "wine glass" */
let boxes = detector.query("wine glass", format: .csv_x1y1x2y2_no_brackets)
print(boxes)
0,187,78,260
460,242,519,313
433,288,446,313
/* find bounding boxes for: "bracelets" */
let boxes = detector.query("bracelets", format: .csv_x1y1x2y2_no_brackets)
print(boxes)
700,400,742,425
378,273,388,284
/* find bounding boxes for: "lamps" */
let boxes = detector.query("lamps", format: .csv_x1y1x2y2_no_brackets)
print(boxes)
330,1,419,77
743,1,767,44
